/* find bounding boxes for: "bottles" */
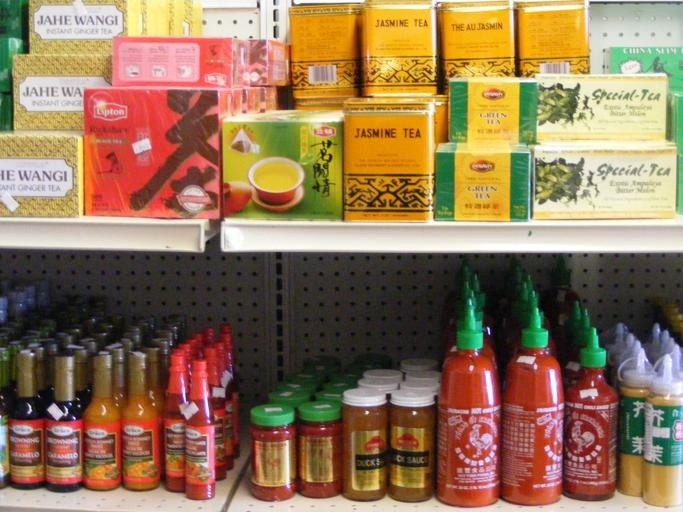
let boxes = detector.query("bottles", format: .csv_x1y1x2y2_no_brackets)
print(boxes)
436,258,683,508
0,280,241,499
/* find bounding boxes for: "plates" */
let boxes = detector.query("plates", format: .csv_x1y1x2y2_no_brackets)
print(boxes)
249,186,304,213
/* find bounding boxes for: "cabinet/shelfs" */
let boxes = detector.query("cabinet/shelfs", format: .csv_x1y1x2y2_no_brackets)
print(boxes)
0,1,683,507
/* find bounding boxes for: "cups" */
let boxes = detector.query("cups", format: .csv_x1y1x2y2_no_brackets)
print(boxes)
248,157,304,206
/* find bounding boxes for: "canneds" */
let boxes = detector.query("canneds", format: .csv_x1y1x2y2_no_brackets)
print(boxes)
264,387,310,407
272,377,318,394
289,369,323,384
295,398,343,500
247,402,299,504
323,379,350,393
314,388,344,405
